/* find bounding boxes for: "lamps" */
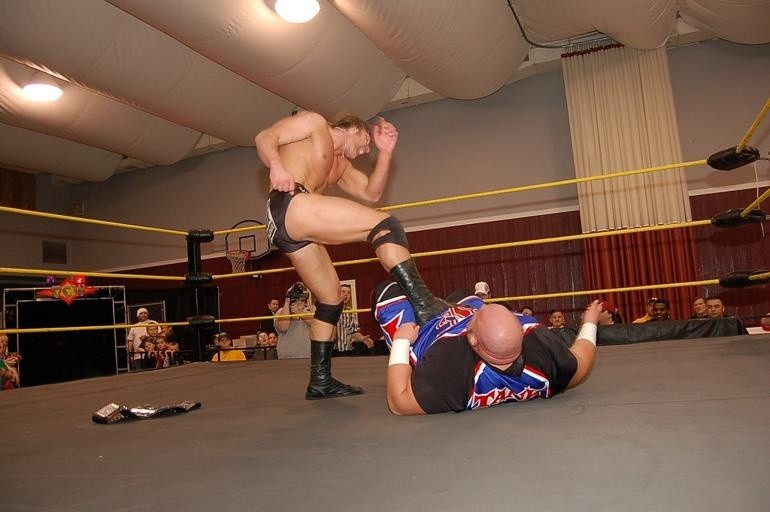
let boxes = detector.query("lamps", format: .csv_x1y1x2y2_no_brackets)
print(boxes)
20,70,64,103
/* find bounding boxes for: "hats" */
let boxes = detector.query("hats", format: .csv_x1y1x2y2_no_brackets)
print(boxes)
474,281,490,295
137,308,148,318
601,302,615,313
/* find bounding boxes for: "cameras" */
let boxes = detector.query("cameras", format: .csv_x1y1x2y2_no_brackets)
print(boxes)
289,286,309,298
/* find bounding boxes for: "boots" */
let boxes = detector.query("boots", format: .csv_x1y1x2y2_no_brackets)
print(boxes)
306,340,364,400
390,258,469,325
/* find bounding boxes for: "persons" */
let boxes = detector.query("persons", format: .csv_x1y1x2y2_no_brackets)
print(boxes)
253,109,457,402
0,339,20,390
125,280,374,370
474,282,726,331
370,271,604,418
0,333,23,367
